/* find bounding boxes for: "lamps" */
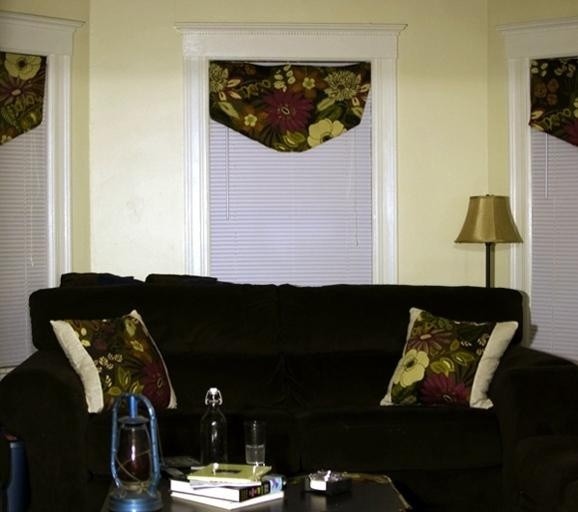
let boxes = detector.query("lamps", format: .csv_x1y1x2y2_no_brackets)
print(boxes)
453,193,521,290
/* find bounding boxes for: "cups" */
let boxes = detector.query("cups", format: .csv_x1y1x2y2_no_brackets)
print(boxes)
242,419,266,465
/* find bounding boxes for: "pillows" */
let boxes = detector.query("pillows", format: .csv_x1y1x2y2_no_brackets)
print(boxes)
48,309,179,417
380,304,518,411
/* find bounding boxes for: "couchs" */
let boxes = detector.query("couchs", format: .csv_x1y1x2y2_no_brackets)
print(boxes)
0,274,576,510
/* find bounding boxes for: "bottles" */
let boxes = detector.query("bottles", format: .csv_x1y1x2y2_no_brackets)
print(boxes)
201,387,229,464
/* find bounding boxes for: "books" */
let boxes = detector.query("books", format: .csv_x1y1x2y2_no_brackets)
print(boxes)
168,461,287,509
